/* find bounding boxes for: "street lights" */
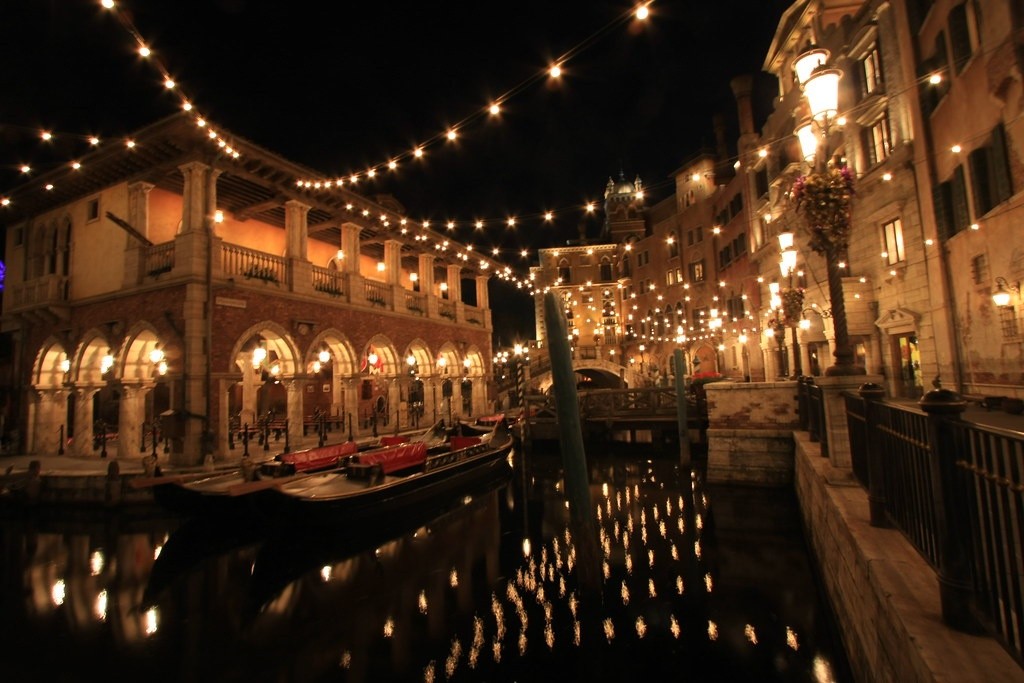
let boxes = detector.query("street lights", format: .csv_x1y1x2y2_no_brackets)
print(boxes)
767,224,799,374
707,306,724,375
793,35,866,376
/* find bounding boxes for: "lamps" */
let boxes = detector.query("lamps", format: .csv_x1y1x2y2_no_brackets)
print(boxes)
800,308,834,330
737,326,762,344
991,277,1021,307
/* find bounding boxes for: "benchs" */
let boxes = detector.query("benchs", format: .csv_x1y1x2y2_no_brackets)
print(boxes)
60,413,390,442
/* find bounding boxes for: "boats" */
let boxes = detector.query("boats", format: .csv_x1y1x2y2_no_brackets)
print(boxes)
176,407,535,510
